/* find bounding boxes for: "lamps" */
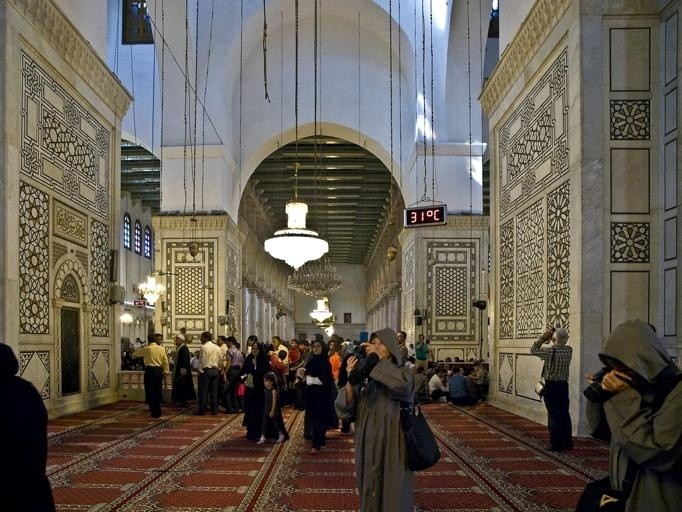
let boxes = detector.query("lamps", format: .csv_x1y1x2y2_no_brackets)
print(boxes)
263,0,344,337
135,0,166,307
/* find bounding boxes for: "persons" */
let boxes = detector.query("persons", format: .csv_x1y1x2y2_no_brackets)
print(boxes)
296,338,340,452
395,331,434,404
334,326,441,512
166,327,196,410
133,333,170,418
0,342,58,511
239,341,290,442
576,318,682,511
428,357,489,407
335,341,364,434
132,338,143,351
530,326,574,452
189,331,366,416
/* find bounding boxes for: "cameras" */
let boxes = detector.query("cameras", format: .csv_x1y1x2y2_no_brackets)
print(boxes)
580,365,625,402
353,345,363,356
545,324,556,335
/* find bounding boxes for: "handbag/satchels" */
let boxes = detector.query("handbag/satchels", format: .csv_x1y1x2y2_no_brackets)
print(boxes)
576,477,627,511
535,380,544,397
400,405,440,470
